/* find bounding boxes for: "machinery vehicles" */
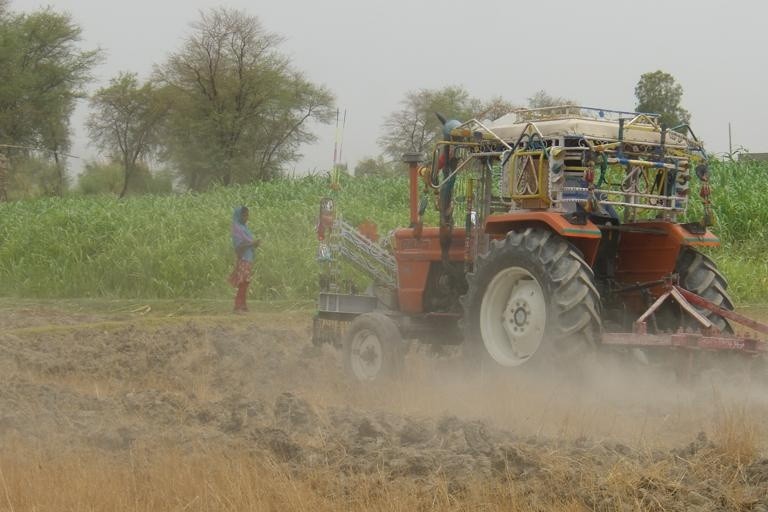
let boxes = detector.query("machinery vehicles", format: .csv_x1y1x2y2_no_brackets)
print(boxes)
317,99,767,391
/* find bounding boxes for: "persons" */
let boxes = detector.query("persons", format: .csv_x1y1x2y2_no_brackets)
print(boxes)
228,206,261,315
